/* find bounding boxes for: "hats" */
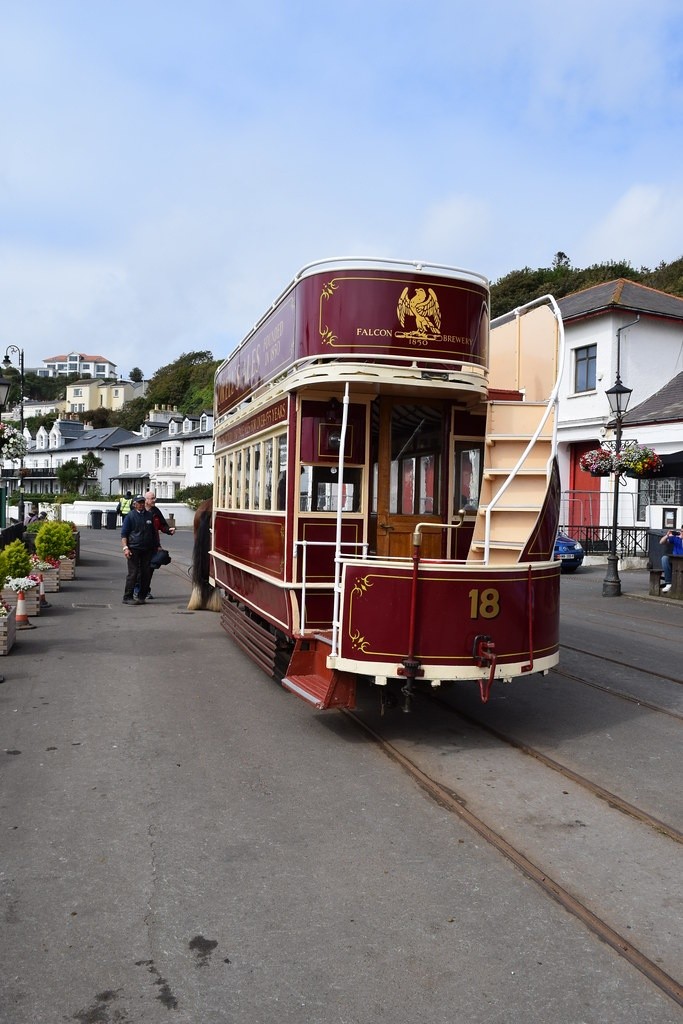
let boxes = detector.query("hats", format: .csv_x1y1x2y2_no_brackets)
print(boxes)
134,494,146,502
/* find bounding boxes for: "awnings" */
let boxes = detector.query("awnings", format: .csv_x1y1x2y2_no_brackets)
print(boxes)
625,450,683,479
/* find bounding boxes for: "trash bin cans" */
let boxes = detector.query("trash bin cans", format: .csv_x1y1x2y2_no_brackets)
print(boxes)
88,510,103,529
105,509,120,530
646,528,678,578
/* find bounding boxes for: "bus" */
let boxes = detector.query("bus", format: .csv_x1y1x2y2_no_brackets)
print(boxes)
208,257,575,717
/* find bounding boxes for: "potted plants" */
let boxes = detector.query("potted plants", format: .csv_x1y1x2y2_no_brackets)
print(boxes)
35,521,65,593
0,538,41,616
57,524,76,580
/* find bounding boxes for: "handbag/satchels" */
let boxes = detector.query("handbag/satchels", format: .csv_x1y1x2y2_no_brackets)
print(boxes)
120,510,122,516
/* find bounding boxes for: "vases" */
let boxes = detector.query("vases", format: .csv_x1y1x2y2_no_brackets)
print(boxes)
0,606,16,657
590,471,610,476
625,470,654,480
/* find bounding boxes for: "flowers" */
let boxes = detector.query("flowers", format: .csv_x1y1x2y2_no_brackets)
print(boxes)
3,574,41,592
0,422,29,463
579,445,665,476
30,550,77,570
0,593,11,617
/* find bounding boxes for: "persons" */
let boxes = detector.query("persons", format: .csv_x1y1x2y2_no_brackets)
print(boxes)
659,525,683,592
23,507,47,532
119,491,175,605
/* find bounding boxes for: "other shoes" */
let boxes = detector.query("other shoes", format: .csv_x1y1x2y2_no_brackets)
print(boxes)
122,597,145,605
145,592,153,599
662,584,672,592
134,592,138,598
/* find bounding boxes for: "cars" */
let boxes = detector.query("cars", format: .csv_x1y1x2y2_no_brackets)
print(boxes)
553,534,584,573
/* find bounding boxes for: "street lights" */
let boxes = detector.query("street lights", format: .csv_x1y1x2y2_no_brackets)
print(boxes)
600,381,633,596
3,343,26,525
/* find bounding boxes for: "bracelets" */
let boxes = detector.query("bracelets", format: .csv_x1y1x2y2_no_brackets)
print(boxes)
123,545,128,551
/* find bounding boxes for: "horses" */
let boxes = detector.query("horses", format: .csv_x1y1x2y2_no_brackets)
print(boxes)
187,496,224,612
